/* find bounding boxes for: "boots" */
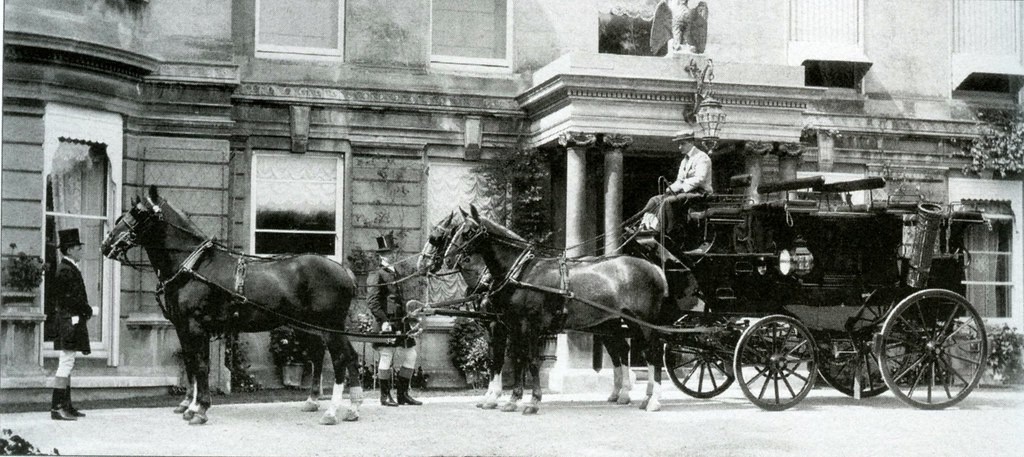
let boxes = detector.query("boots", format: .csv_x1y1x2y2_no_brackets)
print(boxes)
380,379,399,407
397,375,423,405
64,386,86,417
50,388,77,421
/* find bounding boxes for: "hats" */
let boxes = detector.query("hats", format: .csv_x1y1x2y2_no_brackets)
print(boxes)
55,228,86,249
672,129,703,141
375,234,400,252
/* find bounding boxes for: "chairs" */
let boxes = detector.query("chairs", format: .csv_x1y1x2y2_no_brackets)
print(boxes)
689,171,751,245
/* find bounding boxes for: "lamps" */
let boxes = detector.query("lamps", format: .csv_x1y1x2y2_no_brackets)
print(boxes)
682,57,727,154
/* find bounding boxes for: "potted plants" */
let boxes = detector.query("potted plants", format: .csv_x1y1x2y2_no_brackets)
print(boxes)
270,327,325,389
449,314,492,387
1,241,53,311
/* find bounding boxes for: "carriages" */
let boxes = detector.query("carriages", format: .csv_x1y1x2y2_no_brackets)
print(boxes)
100,171,991,428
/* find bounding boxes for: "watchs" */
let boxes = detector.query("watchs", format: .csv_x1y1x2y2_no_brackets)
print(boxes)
50,228,100,421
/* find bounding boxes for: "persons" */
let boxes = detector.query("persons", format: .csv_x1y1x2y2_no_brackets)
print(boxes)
625,128,711,235
366,233,421,407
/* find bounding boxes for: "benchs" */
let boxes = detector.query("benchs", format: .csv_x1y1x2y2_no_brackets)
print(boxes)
743,175,824,250
818,174,886,218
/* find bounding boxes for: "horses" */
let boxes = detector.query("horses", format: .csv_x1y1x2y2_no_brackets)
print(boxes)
107,185,669,425
